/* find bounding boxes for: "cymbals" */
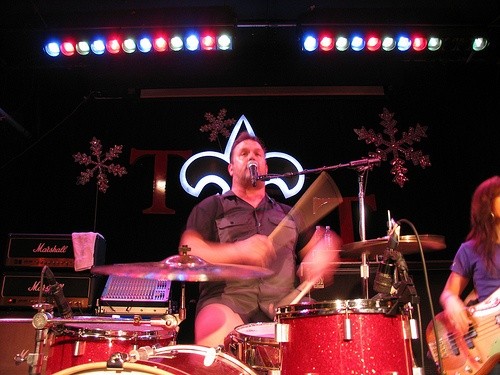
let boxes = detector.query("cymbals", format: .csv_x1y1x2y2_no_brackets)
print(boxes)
92,254,276,282
342,234,446,255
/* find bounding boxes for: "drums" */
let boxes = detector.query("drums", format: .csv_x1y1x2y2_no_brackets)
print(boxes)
222,322,282,375
274,298,419,375
51,344,259,375
36,317,177,375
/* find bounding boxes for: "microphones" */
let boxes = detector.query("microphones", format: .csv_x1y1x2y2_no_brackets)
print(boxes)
372,221,400,294
45,266,73,319
247,160,259,187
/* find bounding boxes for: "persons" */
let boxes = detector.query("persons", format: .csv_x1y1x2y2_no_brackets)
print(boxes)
439,176,500,333
347,270,437,375
178,132,340,350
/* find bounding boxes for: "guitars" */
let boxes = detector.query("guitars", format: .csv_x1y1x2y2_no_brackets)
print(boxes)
425,287,500,375
